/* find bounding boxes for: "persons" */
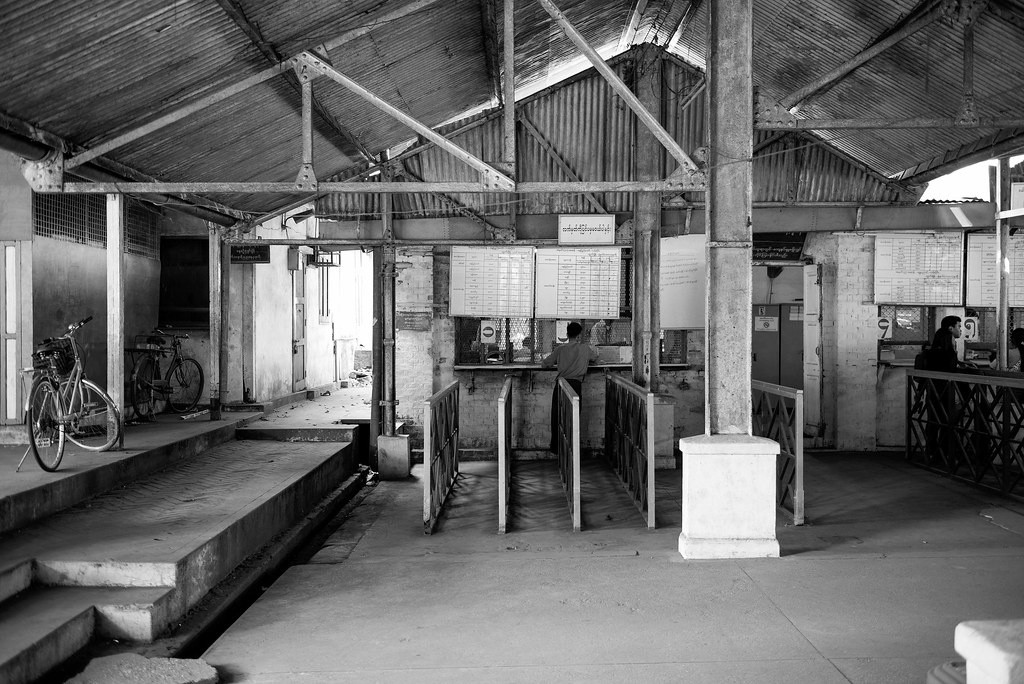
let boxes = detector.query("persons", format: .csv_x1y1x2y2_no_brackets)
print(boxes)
540,322,598,456
514,337,535,358
989,328,1024,404
925,316,977,461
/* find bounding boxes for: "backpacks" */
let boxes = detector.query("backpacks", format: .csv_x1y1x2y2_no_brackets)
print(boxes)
914,351,936,385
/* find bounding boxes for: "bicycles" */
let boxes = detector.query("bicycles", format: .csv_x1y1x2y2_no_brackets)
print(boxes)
15,315,121,474
125,327,204,424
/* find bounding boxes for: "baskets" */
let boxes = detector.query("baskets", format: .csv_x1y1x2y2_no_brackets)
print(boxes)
31,339,75,375
